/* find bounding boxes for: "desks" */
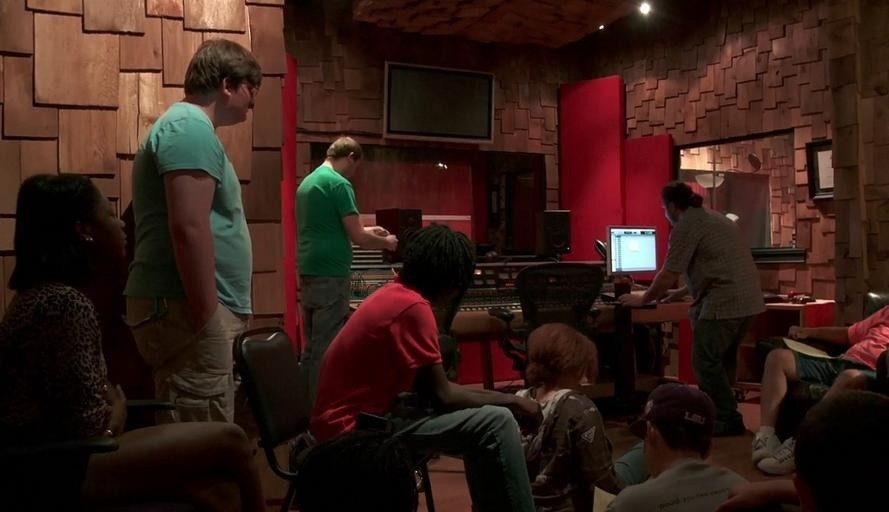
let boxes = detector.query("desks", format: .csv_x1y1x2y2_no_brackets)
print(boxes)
450,297,694,392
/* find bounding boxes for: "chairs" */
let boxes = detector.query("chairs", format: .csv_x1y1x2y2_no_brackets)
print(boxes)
484,259,605,436
0,399,177,512
232,326,436,511
780,285,889,413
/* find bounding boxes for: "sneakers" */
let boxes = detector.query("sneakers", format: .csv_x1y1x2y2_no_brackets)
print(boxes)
756,437,799,477
750,423,782,463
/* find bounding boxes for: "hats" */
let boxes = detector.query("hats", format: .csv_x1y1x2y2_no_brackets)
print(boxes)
627,379,718,442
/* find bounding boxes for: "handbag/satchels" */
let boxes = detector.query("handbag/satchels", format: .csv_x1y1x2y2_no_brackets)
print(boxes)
527,464,576,511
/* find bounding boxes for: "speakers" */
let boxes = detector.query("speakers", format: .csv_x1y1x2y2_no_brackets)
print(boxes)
375,208,423,263
543,210,571,255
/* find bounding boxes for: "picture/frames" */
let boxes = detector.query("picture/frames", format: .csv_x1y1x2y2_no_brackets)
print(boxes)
803,138,838,203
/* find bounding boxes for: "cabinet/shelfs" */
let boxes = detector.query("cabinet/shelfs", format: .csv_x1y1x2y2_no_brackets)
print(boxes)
731,295,839,402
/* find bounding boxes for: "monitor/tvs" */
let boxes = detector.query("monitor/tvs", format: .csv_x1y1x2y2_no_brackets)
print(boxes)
383,61,496,145
607,226,659,276
